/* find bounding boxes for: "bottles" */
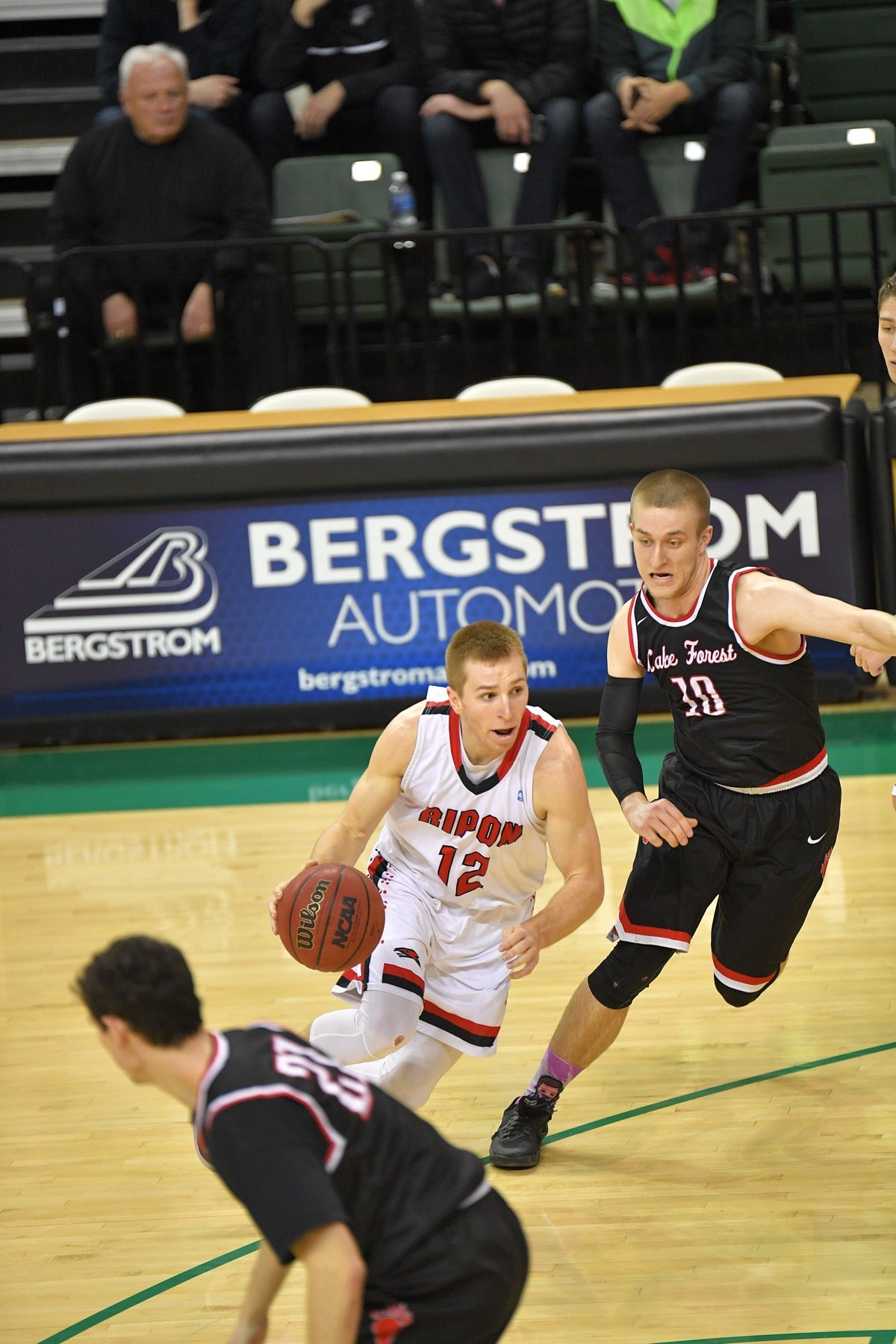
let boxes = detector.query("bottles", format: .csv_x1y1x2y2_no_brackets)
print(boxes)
387,171,420,236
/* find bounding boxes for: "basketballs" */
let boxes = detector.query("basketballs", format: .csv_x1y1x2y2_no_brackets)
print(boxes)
274,863,387,974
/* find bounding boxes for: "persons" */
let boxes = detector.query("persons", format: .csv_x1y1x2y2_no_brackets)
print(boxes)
74,937,530,1344
52,0,779,413
490,470,896,1168
270,620,605,1113
851,273,896,811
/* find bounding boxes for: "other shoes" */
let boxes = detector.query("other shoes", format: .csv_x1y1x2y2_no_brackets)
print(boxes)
608,244,694,285
506,255,547,295
455,255,501,301
684,246,741,293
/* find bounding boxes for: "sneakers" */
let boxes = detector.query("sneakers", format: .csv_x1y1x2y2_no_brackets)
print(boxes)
489,1095,560,1167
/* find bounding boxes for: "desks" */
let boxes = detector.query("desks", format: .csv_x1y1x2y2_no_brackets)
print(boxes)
0,374,896,747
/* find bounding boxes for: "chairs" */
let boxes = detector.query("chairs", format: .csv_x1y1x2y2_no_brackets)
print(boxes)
63,0,896,421
